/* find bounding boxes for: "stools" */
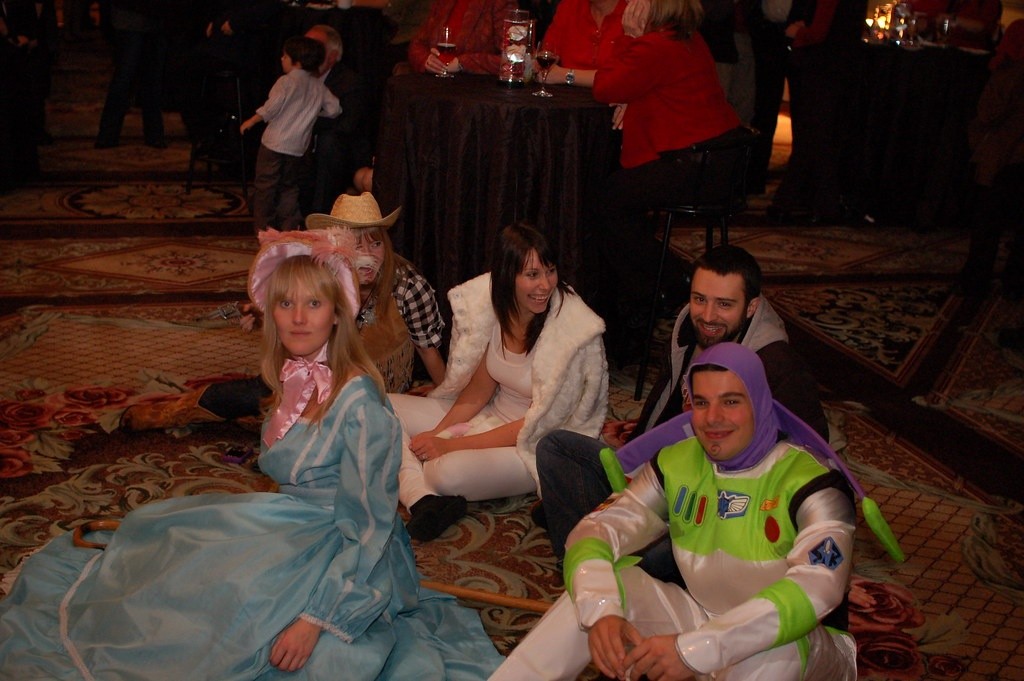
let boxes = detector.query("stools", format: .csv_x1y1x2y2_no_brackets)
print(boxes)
187,63,249,207
634,125,762,401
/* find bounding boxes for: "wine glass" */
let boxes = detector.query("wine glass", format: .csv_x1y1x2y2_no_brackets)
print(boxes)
532,40,559,97
435,25,456,78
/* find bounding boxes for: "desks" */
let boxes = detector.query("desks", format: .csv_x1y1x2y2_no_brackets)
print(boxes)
373,73,623,353
242,5,384,105
776,39,996,233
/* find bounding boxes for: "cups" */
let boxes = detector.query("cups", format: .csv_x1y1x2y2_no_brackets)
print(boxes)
498,8,534,89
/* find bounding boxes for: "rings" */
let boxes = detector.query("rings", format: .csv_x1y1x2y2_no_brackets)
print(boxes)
617,103,623,108
423,453,428,459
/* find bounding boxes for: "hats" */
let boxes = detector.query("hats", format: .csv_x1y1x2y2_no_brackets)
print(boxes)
245,228,362,319
305,191,401,231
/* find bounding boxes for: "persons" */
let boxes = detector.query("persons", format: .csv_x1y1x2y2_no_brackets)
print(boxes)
528,244,830,590
0,225,506,681
407,0,868,317
900,0,1024,352
118,191,446,435
485,343,906,681
0,0,381,245
385,220,609,542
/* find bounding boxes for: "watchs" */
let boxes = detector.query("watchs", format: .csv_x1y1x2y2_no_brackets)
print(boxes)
564,69,576,86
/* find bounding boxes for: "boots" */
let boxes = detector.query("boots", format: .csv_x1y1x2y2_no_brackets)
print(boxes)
118,383,228,432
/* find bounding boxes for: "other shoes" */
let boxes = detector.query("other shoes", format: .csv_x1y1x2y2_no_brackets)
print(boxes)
768,202,822,224
408,494,468,542
92,129,170,150
